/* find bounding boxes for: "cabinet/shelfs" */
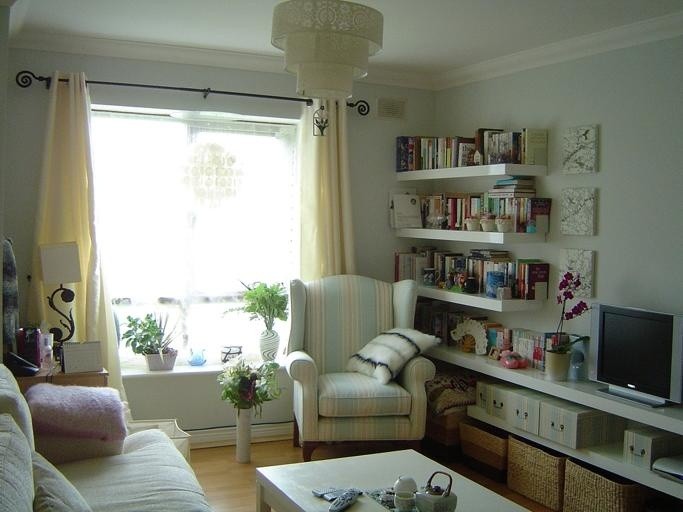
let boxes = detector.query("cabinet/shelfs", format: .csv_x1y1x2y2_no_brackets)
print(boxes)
117,359,305,434
424,344,681,503
390,125,556,315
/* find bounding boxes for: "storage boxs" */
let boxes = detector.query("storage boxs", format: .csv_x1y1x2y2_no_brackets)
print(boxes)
471,376,678,475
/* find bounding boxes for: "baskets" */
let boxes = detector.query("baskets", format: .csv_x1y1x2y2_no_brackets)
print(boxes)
458,418,509,471
425,403,473,447
506,433,568,512
562,456,648,512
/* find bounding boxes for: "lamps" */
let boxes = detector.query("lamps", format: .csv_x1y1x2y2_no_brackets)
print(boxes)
37,240,82,359
267,0,383,138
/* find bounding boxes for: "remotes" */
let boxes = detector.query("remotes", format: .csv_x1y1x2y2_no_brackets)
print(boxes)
324,488,347,501
328,489,360,511
312,484,336,497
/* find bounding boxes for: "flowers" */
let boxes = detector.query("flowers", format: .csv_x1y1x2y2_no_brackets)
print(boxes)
216,358,282,420
544,269,591,353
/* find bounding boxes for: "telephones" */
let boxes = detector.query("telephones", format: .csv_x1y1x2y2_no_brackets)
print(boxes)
3,352,39,376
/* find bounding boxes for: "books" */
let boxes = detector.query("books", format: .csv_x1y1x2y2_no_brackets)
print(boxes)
414,302,570,373
389,175,551,232
394,247,548,299
395,128,548,171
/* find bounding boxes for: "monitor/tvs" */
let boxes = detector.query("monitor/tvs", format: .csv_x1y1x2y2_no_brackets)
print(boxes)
595,303,674,409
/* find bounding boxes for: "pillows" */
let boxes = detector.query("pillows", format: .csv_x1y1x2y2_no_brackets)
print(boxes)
21,379,129,435
0,363,92,512
32,431,129,458
340,323,447,386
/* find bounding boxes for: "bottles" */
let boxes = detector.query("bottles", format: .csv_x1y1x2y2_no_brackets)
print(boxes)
464,276,476,293
40,334,53,370
488,141,506,164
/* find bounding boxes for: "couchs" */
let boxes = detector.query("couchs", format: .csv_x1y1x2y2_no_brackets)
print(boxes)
287,270,439,467
0,360,223,512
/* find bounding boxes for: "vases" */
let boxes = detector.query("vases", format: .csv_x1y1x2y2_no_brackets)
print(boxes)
234,406,253,463
544,349,575,383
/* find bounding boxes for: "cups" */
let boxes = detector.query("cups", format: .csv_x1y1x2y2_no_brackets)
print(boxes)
422,268,440,286
394,473,417,494
496,287,510,300
485,271,504,298
393,490,416,511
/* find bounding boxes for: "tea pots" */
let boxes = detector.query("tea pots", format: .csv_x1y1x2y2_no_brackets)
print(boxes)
415,471,458,512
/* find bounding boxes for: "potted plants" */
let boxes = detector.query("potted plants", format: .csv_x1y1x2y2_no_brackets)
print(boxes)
232,279,293,364
123,307,184,378
